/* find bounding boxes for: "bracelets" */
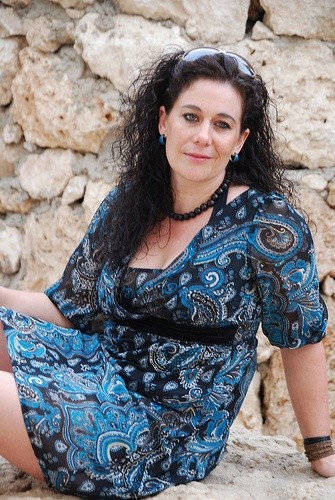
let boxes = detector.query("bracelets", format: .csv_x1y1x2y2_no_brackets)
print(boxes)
302,436,335,462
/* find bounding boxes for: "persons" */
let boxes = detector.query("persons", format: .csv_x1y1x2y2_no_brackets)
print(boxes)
0,48,335,500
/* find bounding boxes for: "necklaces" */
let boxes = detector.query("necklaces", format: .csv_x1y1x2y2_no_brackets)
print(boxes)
166,168,231,221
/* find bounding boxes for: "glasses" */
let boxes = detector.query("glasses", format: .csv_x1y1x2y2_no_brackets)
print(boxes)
178,47,257,83
